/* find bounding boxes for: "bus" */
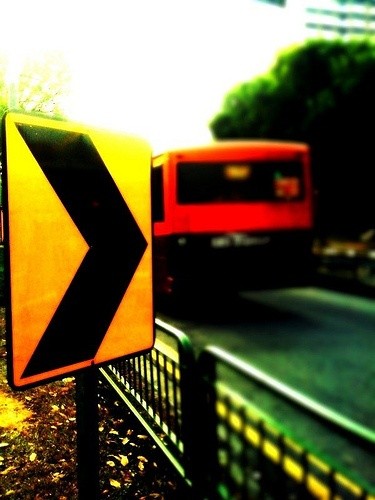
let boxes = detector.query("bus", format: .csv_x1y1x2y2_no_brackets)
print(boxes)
151,138,311,313
